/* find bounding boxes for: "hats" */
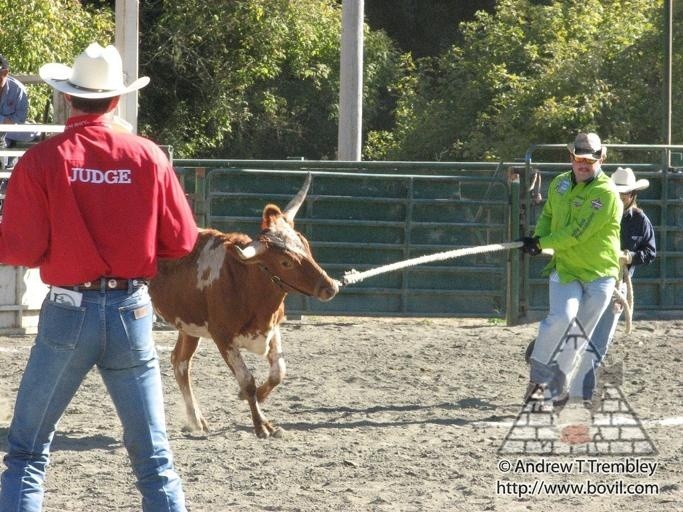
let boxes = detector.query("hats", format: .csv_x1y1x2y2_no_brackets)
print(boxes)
39,41,151,100
610,167,651,194
566,133,607,161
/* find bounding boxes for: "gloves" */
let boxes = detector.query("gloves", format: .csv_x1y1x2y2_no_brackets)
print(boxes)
620,250,632,265
514,235,541,256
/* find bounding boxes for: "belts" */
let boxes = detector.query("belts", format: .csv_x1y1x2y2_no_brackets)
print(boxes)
64,275,142,291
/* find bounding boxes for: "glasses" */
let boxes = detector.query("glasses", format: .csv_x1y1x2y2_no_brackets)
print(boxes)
572,154,598,164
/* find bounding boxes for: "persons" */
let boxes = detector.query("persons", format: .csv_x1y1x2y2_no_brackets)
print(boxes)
514,132,623,406
567,166,657,407
0,42,199,512
0,55,29,210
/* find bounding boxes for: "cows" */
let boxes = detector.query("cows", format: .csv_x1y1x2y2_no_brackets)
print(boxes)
528,326,624,443
146,171,340,439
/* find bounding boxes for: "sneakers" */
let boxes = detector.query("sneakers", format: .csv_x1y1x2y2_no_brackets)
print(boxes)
526,379,593,410
